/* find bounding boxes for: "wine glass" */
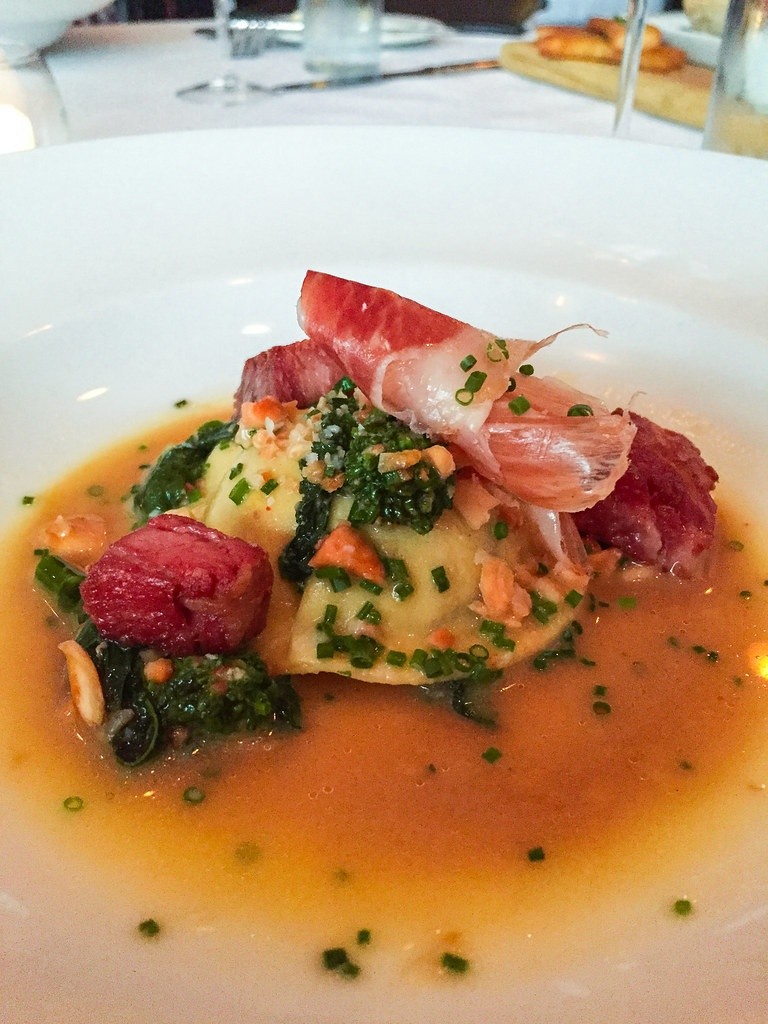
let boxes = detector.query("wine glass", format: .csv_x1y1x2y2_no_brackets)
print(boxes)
611,0,649,139
176,1,271,106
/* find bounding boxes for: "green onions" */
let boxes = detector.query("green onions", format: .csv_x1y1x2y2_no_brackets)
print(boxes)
15,337,767,986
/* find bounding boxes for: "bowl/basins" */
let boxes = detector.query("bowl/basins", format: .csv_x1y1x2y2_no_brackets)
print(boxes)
644,11,767,69
0,0,114,63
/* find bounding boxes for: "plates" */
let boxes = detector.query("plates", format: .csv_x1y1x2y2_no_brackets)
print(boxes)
0,122,765,1024
269,15,442,46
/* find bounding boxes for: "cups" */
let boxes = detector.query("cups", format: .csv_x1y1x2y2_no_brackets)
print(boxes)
701,0,768,160
298,0,384,78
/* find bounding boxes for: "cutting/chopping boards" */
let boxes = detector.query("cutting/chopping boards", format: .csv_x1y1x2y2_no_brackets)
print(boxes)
498,42,768,159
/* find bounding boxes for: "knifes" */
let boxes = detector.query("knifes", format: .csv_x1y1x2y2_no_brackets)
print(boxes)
272,58,502,93
